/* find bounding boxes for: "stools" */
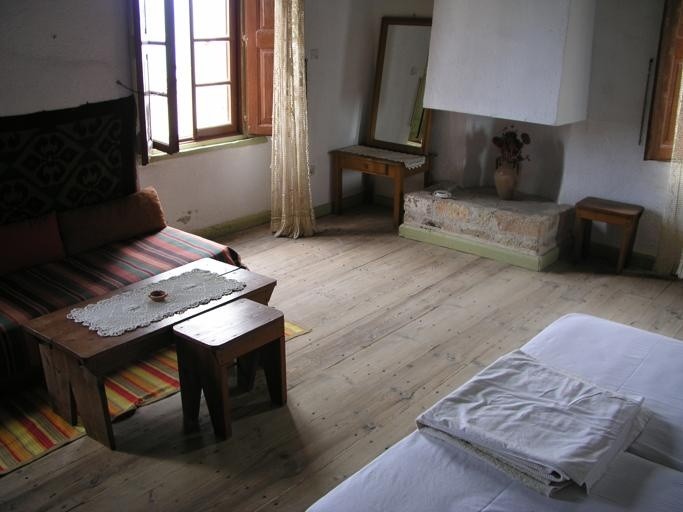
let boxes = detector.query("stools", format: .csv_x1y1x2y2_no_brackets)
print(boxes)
573,195,645,273
173,297,287,440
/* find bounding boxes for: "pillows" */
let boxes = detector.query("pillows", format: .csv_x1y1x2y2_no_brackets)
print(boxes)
56,185,166,257
0,210,67,274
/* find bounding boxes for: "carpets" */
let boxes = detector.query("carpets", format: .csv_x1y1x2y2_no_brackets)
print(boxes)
0,299,312,477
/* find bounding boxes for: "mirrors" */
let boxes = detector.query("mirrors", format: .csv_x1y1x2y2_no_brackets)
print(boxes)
368,15,436,156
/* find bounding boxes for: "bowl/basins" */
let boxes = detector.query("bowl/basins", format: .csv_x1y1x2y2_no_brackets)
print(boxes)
148,289,168,302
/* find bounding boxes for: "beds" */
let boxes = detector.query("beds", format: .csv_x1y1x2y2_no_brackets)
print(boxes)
306,313,682,510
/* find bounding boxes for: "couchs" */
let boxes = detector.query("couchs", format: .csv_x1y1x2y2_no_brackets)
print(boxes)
0,192,246,397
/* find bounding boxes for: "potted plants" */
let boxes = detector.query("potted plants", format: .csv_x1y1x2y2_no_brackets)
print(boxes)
492,125,532,200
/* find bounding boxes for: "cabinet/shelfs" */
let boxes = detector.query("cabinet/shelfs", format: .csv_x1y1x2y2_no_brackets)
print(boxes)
328,145,437,229
